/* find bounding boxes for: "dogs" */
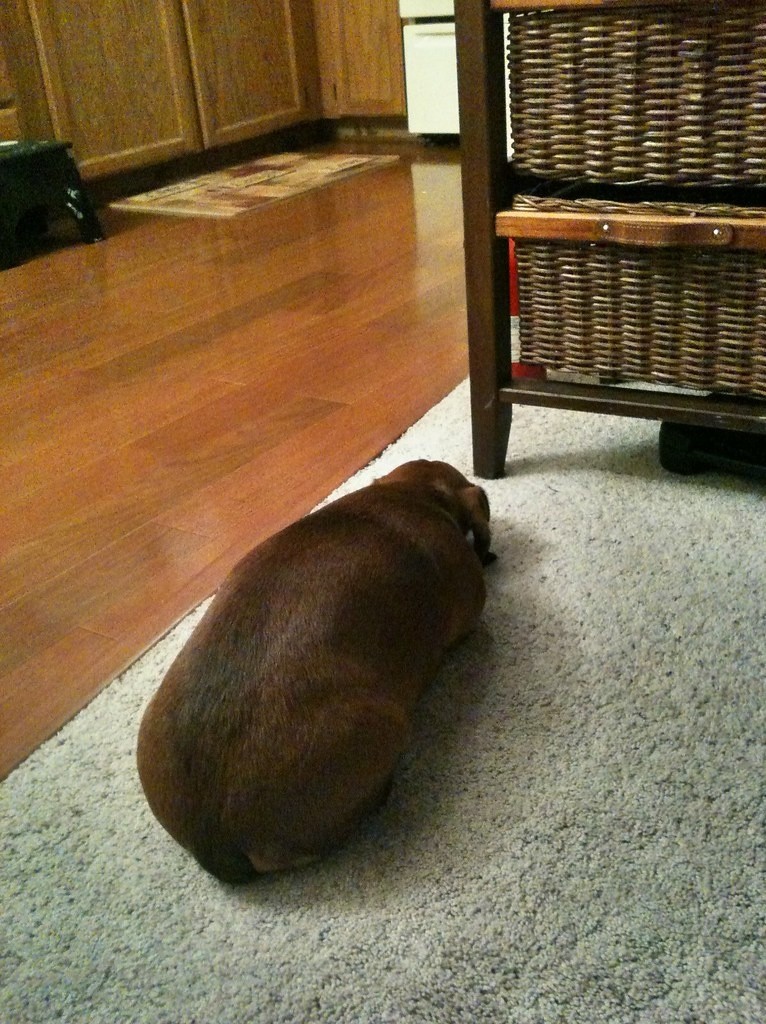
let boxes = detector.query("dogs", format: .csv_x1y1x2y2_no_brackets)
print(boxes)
136,458,494,878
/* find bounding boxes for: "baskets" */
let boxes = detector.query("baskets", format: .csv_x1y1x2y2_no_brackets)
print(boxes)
508,0,766,188
511,194,766,401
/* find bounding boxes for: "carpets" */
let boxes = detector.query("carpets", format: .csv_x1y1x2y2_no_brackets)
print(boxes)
0,313,766,1024
94,151,400,218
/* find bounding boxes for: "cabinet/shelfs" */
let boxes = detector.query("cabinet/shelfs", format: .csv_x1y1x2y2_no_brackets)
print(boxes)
455,0,766,480
0,0,407,181
